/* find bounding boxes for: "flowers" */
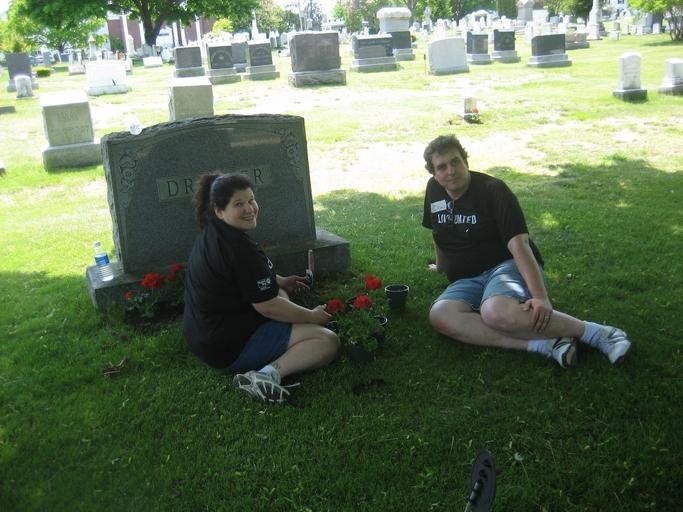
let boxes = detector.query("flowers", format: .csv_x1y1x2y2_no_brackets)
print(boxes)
324,274,385,353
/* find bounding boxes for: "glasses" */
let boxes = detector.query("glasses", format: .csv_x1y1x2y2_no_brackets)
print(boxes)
446,201,454,225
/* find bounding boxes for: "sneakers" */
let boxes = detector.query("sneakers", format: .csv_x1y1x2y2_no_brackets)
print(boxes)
551,336,583,368
232,370,290,403
589,325,632,367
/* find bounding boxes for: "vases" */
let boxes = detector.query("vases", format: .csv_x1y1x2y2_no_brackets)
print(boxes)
343,335,375,365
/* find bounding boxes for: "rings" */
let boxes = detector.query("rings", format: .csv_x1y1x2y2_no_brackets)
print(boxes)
544,316,549,321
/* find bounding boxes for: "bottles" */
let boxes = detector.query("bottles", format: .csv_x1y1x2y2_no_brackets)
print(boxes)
93,241,114,282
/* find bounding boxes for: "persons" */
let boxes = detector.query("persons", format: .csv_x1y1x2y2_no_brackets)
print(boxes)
180,173,342,404
422,135,633,372
